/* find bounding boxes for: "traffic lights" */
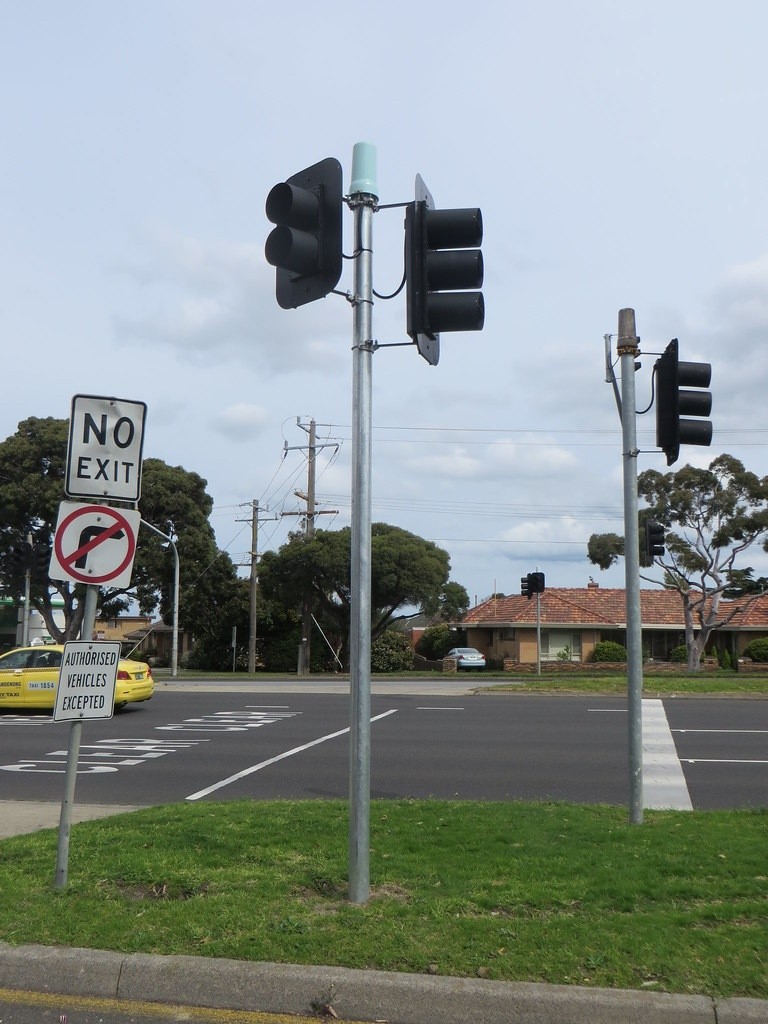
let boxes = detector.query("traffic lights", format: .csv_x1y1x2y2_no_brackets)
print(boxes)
645,520,665,557
655,338,712,466
264,158,342,309
531,573,545,593
521,573,532,600
406,173,485,366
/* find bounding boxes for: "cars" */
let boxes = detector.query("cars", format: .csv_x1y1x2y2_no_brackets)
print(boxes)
0,645,154,709
448,648,486,672
